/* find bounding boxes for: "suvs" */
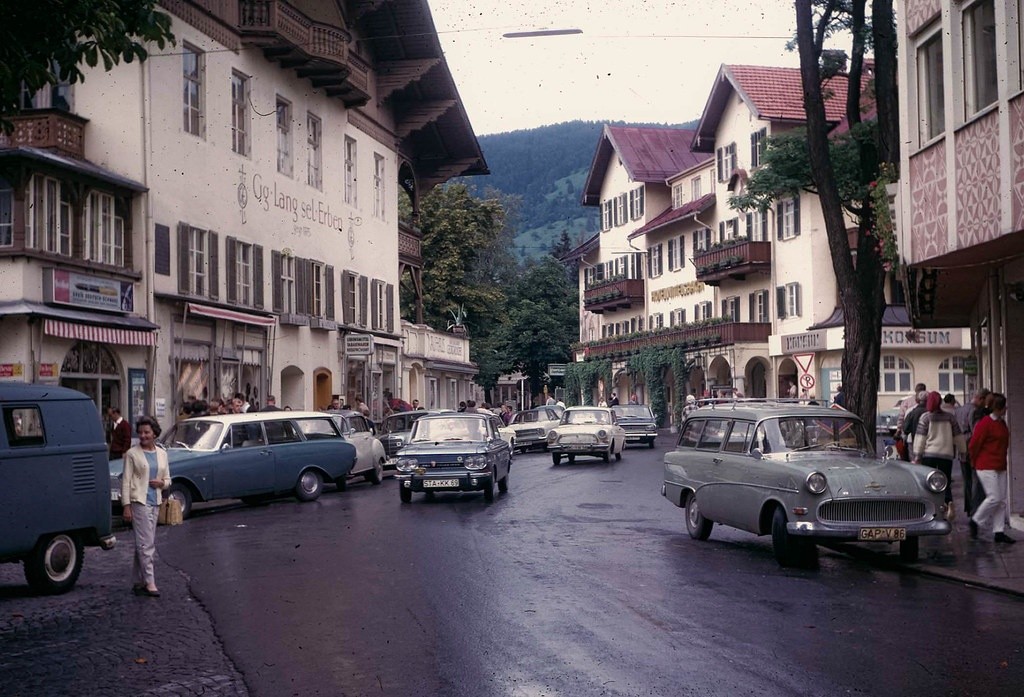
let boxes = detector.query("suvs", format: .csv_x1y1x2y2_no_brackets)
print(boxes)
661,397,952,573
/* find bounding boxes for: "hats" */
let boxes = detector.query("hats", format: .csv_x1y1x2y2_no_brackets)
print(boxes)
919,391,928,400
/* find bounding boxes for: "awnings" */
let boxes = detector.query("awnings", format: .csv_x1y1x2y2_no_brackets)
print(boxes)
154,291,282,317
0,304,159,344
338,323,408,339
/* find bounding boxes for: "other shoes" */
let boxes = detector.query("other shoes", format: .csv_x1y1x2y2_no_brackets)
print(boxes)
947,502,956,521
993,533,1017,544
969,520,979,539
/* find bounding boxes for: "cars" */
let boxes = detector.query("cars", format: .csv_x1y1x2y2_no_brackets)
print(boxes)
876,395,962,436
393,412,512,504
0,380,118,596
611,404,658,448
463,414,517,459
284,410,388,492
534,405,565,419
108,411,357,520
363,409,466,481
507,409,561,454
546,406,626,465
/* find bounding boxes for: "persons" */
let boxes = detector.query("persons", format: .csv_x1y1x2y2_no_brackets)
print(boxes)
392,399,424,425
467,420,481,439
457,400,512,425
326,397,369,417
897,382,1016,543
177,393,292,445
808,395,819,405
121,416,171,596
598,393,619,408
834,386,841,405
788,380,797,397
109,408,132,460
631,392,637,404
681,388,743,421
546,394,566,410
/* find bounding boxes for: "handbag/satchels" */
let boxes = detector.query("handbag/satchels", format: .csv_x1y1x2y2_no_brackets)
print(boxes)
157,485,184,526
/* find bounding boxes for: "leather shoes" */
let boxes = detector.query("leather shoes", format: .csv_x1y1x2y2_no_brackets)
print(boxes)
133,584,147,595
144,585,160,596
